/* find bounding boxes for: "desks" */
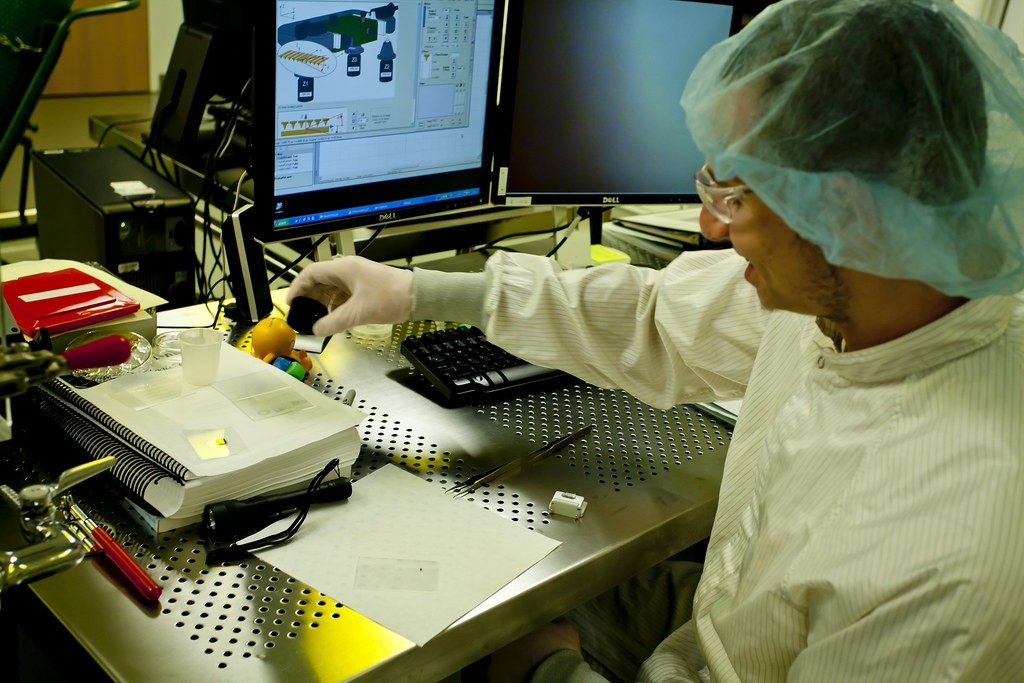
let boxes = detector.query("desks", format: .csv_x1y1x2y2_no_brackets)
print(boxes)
0,222,749,683
89,112,579,276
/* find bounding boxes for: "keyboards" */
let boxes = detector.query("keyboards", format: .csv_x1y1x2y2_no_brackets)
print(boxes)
399,324,570,406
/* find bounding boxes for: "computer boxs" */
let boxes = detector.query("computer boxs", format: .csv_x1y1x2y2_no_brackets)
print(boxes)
31,144,195,313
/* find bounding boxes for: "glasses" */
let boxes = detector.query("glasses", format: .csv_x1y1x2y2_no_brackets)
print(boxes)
695,163,751,224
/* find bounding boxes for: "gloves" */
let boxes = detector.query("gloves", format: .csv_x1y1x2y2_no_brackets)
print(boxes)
487,612,580,683
286,255,414,338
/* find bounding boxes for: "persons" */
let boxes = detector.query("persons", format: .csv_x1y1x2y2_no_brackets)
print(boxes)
283,0,1024,683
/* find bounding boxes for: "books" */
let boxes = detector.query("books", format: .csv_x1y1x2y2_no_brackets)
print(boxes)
20,340,363,533
609,207,716,254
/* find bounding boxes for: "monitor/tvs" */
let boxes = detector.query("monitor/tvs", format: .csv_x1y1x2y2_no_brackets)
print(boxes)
255,0,500,241
151,0,258,166
490,0,743,245
